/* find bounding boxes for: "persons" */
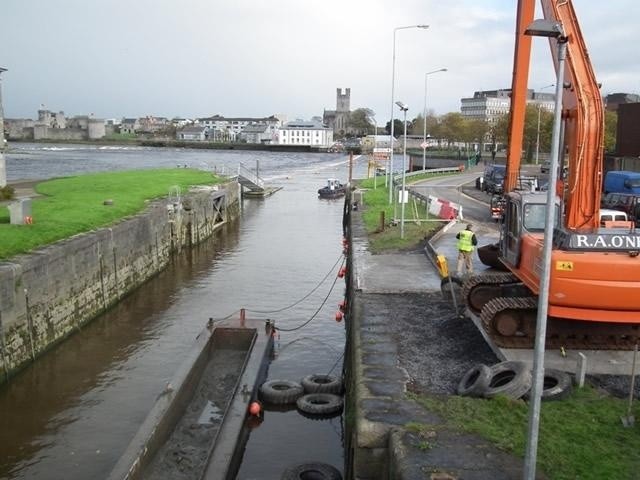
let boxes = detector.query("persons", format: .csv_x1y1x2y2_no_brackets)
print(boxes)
454,223,478,276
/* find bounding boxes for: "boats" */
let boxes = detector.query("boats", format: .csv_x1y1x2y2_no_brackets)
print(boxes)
316,178,355,200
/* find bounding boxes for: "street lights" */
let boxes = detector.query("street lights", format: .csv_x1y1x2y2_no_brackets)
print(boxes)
535,83,556,163
422,68,447,174
387,22,431,207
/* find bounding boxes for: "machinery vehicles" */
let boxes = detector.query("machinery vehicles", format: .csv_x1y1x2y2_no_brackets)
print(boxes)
462,0,640,355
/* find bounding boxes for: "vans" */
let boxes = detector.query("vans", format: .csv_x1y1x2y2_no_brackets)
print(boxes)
476,155,640,229
